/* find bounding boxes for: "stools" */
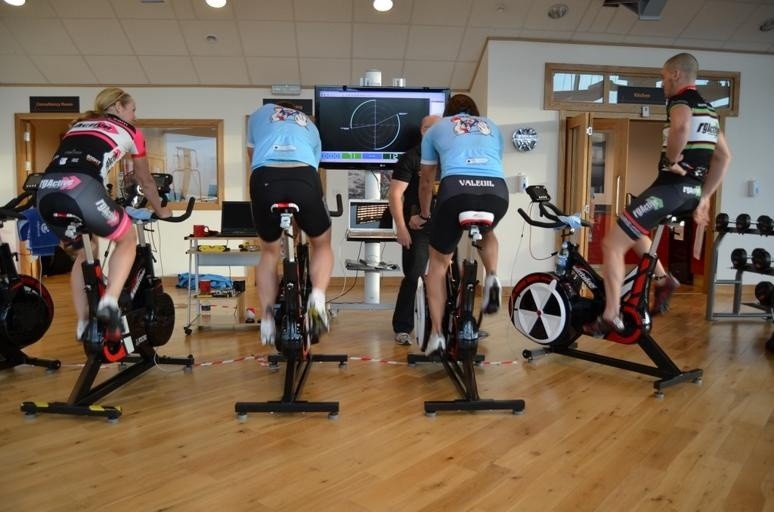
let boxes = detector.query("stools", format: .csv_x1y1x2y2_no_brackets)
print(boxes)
192,290,245,332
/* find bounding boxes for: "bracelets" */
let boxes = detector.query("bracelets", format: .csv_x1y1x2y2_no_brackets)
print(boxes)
419,213,432,220
660,156,676,167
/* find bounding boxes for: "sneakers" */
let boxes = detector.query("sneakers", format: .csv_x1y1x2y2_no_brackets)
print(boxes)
652,272,681,316
424,331,446,358
305,288,330,336
581,312,626,335
75,318,105,344
393,332,413,346
95,295,130,364
258,318,279,347
482,274,503,316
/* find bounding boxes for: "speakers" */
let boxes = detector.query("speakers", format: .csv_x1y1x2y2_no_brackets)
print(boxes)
271,85,301,95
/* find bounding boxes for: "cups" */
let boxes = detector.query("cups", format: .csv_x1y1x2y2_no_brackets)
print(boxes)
392,78,406,88
200,280,211,293
193,224,209,236
363,68,381,86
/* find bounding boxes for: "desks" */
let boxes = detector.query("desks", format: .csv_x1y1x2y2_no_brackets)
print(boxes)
182,231,260,337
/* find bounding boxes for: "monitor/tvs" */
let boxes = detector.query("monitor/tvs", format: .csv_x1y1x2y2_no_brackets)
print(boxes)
315,85,451,171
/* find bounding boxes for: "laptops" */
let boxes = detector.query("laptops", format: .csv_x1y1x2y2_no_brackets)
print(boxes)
348,199,395,235
216,201,257,237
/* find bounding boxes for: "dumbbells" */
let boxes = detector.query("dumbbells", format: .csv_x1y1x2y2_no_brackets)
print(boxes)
717,213,774,305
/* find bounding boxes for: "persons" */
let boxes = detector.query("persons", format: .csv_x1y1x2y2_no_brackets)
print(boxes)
386,114,460,346
245,101,334,348
408,94,510,359
35,90,172,343
578,52,730,334
